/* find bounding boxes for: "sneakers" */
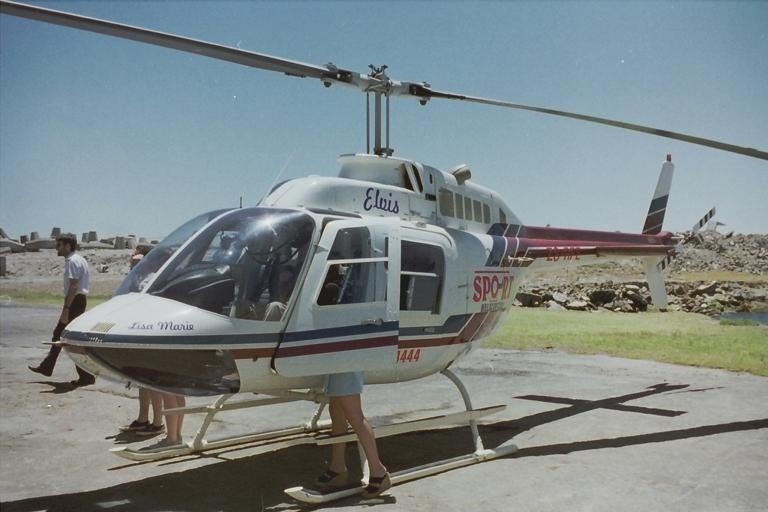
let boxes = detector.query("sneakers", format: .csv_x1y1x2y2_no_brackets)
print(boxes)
118,420,183,449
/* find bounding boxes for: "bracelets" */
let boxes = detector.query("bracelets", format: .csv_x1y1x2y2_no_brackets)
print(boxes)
64,305,70,309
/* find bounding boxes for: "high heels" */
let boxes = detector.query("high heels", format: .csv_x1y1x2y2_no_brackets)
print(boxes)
360,471,392,498
314,464,350,490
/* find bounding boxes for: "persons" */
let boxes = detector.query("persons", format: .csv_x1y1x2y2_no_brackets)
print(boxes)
140,245,185,449
229,240,307,321
27,233,96,388
130,242,143,255
119,254,165,437
301,265,392,499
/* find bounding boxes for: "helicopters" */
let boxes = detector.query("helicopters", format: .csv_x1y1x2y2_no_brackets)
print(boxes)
0,0,768,506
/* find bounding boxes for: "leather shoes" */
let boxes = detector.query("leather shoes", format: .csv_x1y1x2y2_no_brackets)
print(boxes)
28,365,95,387
